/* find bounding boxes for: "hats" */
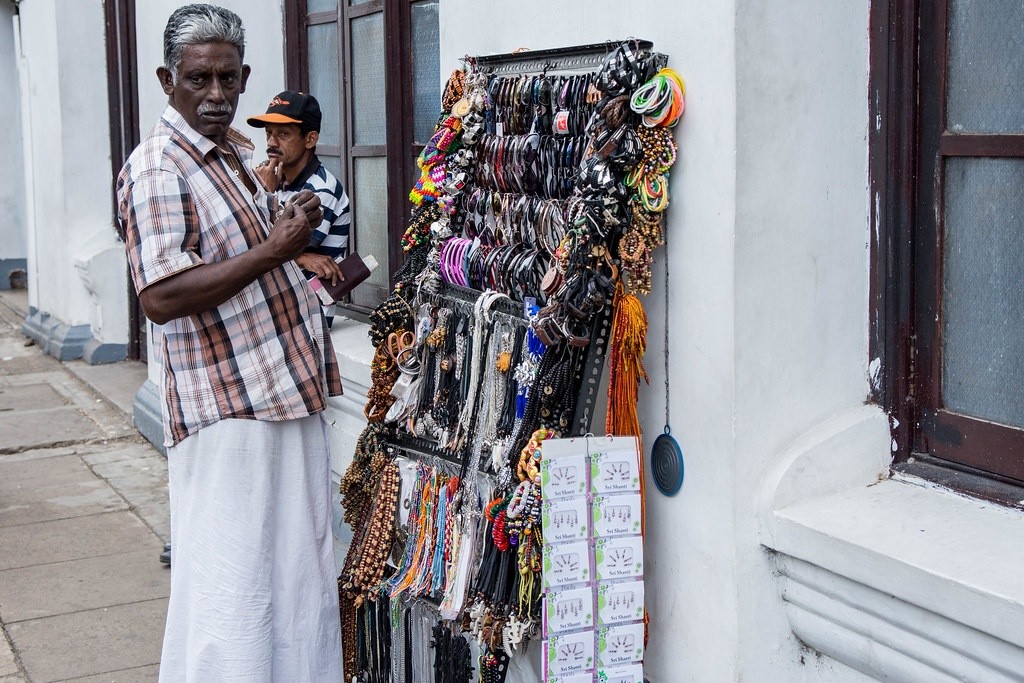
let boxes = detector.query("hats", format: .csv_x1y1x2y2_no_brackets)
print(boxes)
247,91,322,132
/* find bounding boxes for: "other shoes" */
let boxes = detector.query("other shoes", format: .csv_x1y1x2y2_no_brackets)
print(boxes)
160,543,172,563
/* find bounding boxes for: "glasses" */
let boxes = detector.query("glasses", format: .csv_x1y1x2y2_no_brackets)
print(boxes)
438,74,598,308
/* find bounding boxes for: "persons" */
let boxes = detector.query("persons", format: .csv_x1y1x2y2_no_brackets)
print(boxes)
161,89,352,569
116,4,345,681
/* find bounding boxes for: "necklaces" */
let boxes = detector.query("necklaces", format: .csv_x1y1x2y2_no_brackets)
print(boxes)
504,337,582,485
340,289,532,683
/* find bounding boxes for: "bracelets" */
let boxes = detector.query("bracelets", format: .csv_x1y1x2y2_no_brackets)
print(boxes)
484,428,563,620
473,288,514,323
484,40,688,347
335,61,478,531
